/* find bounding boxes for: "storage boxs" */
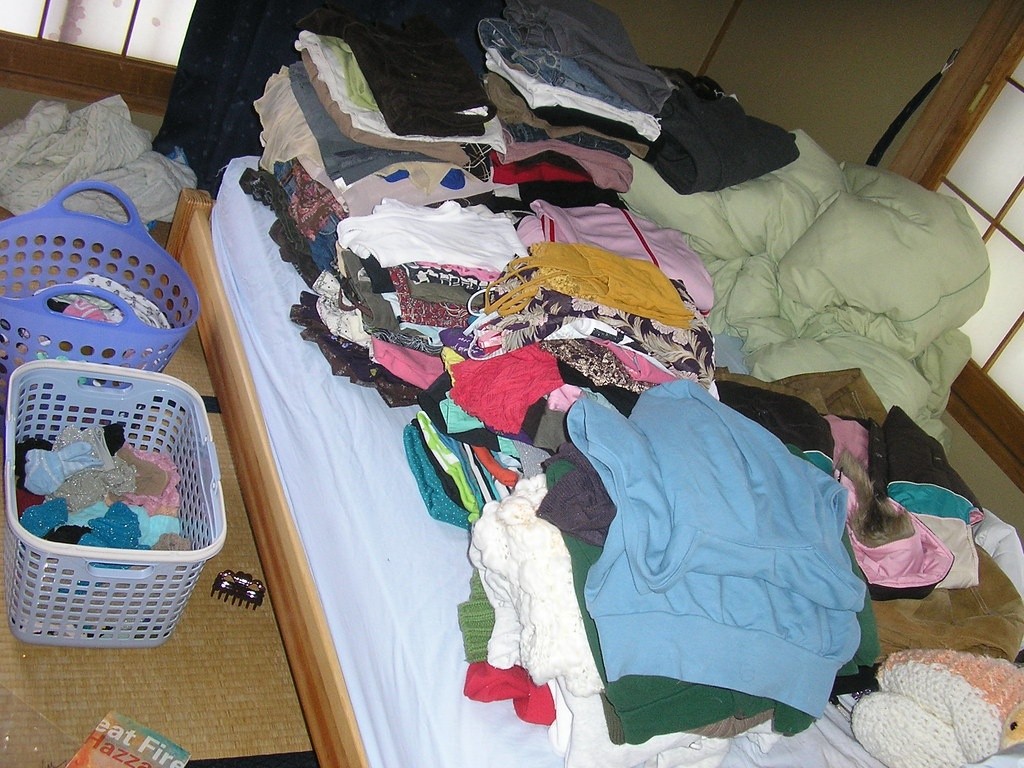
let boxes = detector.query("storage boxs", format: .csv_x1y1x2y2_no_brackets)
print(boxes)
3,359,230,649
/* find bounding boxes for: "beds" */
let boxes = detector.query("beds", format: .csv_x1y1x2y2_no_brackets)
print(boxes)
165,154,1024,768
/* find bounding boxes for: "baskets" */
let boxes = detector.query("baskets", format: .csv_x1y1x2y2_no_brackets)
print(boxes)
5,359,227,647
0,179,200,440
